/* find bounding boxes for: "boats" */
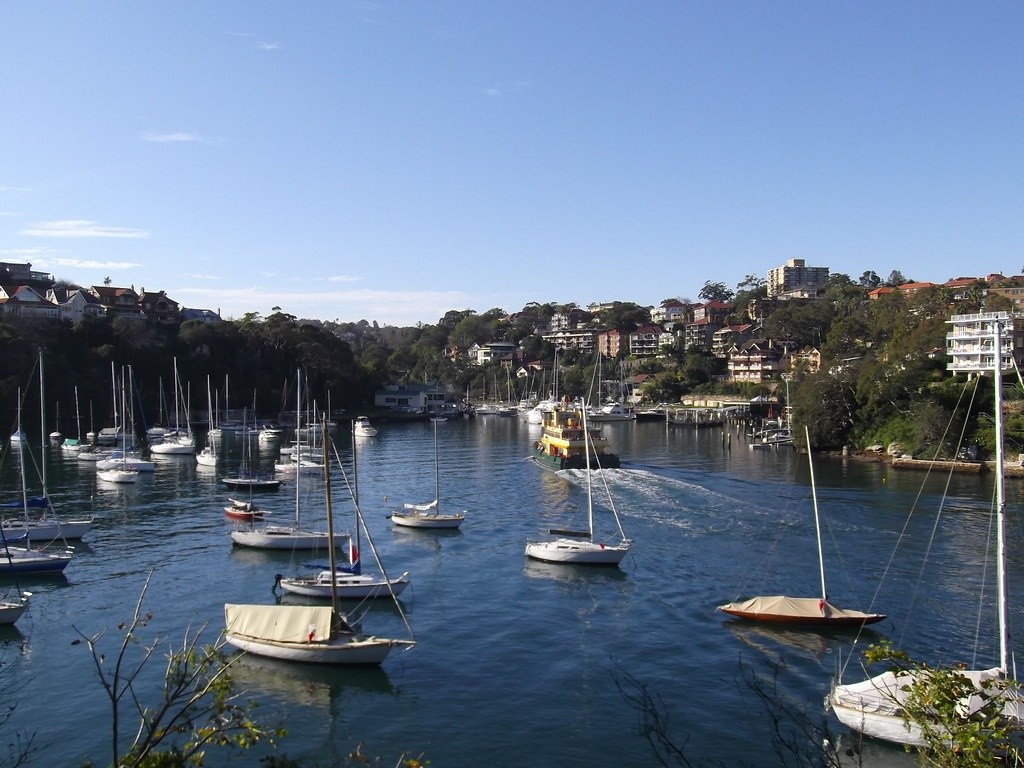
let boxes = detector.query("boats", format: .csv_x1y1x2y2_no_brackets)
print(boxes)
530,397,621,474
10,429,28,441
350,415,378,437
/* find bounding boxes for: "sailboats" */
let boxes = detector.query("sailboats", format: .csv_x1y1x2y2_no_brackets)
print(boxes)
96,365,139,484
272,434,413,599
220,409,420,665
465,340,732,426
0,386,76,573
48,401,62,440
383,420,468,529
715,424,888,629
0,522,33,627
523,397,635,566
223,432,271,519
228,364,355,552
60,356,338,491
0,346,98,540
825,312,1024,759
